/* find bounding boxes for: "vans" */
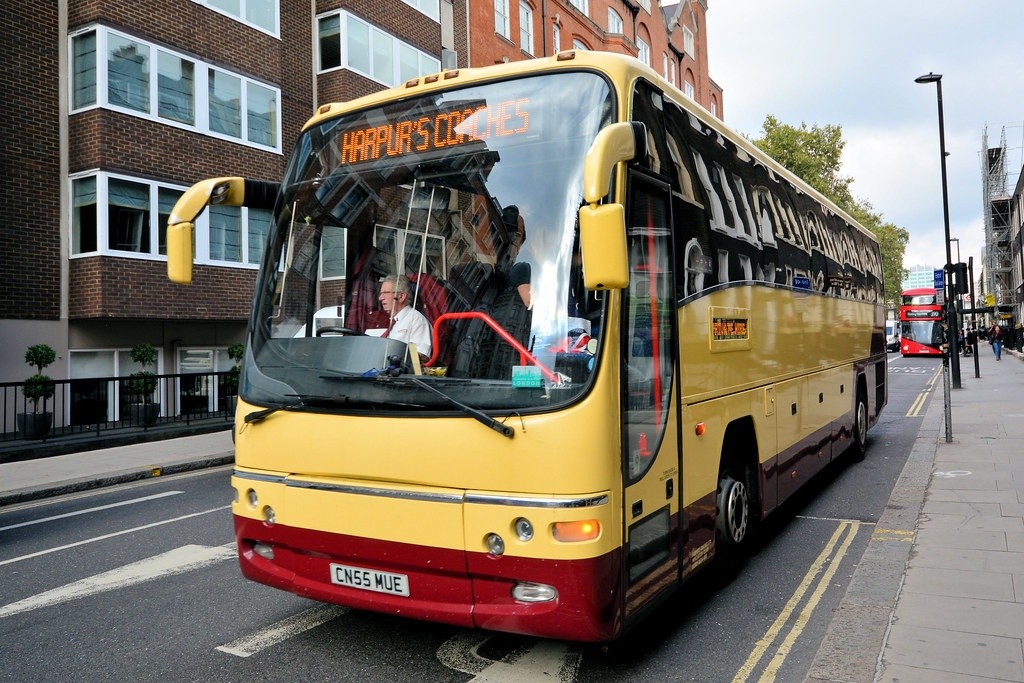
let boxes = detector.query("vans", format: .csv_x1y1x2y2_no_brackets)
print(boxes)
886,320,901,352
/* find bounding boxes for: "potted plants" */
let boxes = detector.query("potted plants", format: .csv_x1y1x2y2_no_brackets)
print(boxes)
17,344,55,438
224,344,244,416
127,342,162,426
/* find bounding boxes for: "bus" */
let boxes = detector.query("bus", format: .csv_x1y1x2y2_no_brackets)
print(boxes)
897,288,944,357
168,50,889,644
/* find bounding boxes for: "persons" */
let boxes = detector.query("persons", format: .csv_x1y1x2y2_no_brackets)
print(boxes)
988,325,1003,361
957,328,974,354
377,275,434,362
977,326,992,343
511,220,560,311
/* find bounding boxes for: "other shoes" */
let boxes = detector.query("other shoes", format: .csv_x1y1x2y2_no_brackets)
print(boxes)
968,351,973,353
996,358,999,361
965,351,968,354
959,353,962,355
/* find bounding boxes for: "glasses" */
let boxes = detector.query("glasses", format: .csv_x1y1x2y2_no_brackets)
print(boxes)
378,291,403,297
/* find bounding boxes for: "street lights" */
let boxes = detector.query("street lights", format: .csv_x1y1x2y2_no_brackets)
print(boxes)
950,238,960,264
914,72,960,389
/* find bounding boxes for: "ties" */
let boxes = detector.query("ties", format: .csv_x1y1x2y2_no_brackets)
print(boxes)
380,319,396,338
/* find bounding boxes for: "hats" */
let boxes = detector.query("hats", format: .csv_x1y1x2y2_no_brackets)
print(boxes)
995,326,1000,330
966,327,970,331
